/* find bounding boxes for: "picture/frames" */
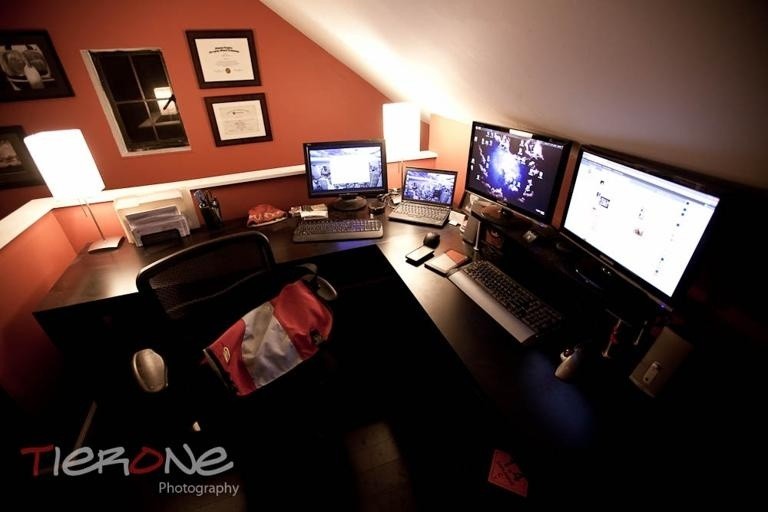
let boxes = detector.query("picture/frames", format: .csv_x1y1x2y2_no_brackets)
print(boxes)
0,25,78,108
183,25,264,89
1,120,43,194
201,92,276,148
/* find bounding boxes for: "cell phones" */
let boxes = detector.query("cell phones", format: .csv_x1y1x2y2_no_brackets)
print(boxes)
405,245,434,262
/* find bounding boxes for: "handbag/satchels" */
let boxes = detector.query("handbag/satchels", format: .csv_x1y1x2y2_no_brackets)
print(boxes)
203,279,334,399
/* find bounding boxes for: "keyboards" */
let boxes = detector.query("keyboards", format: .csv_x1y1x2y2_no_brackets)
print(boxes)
292,218,384,243
446,259,562,344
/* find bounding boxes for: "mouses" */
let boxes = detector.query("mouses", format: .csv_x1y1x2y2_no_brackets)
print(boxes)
423,231,440,247
553,341,585,383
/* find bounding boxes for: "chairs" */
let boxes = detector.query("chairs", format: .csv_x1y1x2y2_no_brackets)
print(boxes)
127,230,341,420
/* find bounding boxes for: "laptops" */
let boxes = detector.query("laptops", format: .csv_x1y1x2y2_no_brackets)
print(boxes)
388,166,458,228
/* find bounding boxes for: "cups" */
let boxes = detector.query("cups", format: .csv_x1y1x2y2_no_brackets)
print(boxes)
199,200,223,231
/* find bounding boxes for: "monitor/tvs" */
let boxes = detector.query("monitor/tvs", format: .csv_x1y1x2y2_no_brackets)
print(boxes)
553,144,725,309
464,120,573,234
302,138,388,212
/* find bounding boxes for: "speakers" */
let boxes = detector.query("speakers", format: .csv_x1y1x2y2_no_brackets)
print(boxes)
629,325,692,398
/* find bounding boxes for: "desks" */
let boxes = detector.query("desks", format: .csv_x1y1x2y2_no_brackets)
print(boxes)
379,209,707,491
26,187,453,416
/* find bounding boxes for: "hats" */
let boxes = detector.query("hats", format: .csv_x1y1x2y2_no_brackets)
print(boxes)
246,204,287,227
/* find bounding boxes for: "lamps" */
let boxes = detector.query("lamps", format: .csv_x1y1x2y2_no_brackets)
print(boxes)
380,101,425,209
154,85,180,120
20,127,122,253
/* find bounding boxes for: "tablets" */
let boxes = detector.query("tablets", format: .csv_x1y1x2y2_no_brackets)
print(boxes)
424,248,472,277
